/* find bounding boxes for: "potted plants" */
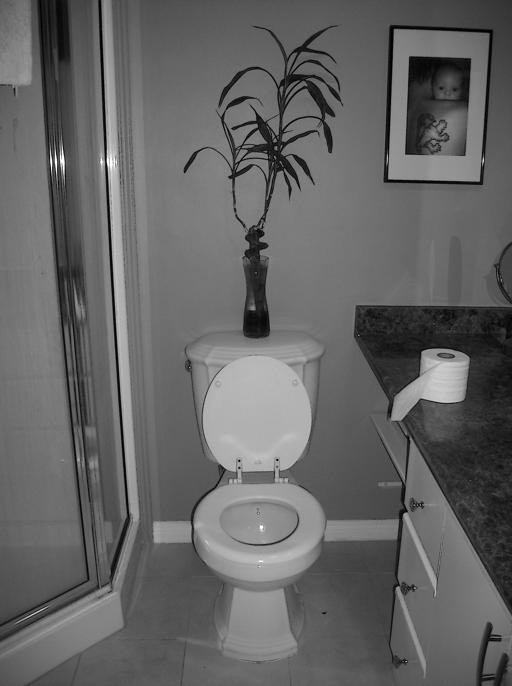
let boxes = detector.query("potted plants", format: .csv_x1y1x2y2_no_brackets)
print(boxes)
185,24,349,341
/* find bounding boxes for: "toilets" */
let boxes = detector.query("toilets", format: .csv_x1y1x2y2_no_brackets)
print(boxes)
184,331,326,663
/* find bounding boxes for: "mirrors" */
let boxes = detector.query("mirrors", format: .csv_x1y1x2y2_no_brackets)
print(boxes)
494,241,511,339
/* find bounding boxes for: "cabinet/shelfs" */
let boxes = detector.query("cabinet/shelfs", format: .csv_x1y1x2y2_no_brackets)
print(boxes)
370,406,408,483
425,445,512,685
390,431,446,686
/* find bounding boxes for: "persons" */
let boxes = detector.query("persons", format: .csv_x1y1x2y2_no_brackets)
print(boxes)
430,64,465,101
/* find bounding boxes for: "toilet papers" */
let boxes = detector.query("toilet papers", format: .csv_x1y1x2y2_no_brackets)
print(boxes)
389,347,472,423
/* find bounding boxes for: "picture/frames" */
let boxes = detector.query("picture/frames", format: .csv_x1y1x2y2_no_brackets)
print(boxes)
380,23,494,187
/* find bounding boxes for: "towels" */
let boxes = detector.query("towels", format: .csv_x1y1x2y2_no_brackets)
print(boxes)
0,0,34,87
